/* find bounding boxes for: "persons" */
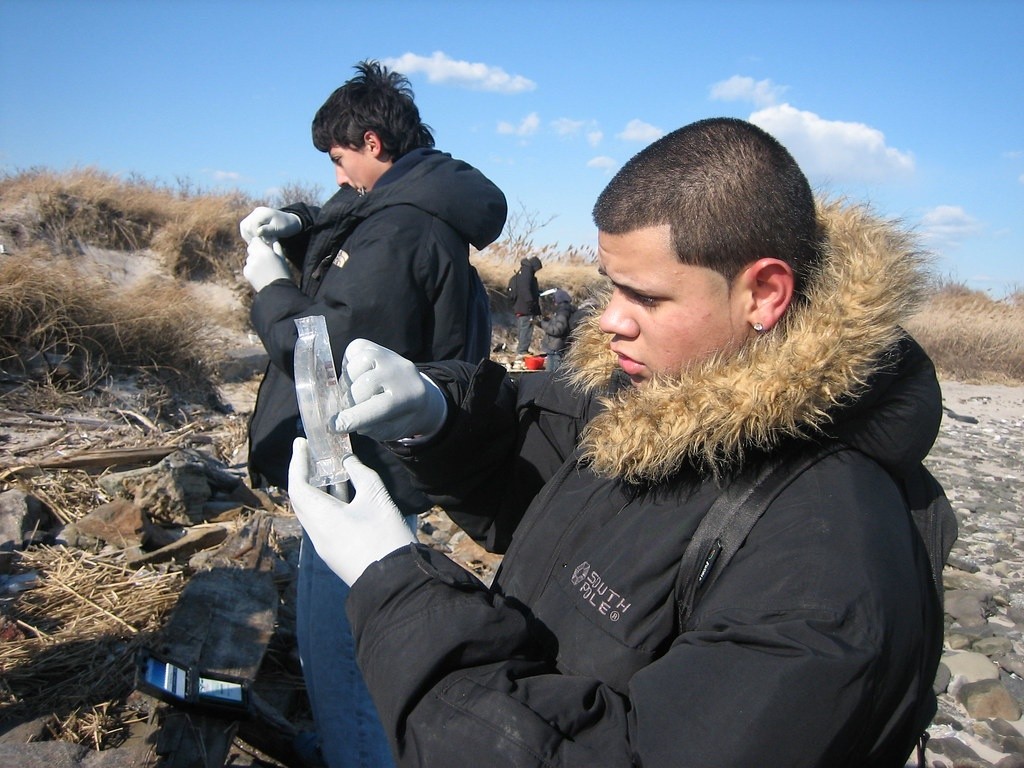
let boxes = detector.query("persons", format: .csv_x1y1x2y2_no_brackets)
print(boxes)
240,56,509,768
511,254,579,369
286,117,957,767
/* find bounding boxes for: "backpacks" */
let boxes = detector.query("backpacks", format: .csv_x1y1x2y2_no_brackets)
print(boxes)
671,438,960,768
505,274,519,303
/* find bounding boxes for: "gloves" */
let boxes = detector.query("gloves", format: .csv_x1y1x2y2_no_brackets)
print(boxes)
288,436,419,589
243,238,289,291
240,206,301,243
334,337,447,445
